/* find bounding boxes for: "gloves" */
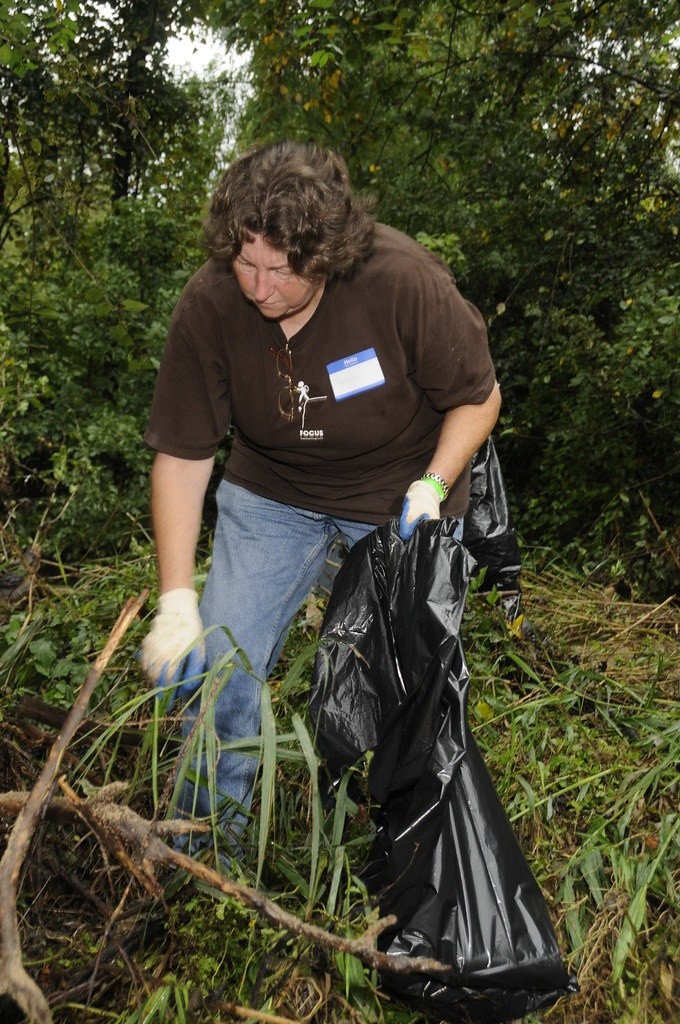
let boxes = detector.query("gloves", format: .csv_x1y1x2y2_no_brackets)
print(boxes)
140,588,205,712
398,479,445,541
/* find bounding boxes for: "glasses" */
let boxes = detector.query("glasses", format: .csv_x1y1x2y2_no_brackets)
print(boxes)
270,342,295,422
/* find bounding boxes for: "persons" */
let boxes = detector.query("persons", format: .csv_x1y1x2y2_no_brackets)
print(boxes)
137,137,503,871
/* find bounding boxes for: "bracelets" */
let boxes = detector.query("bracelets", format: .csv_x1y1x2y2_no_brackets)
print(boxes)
420,473,449,503
